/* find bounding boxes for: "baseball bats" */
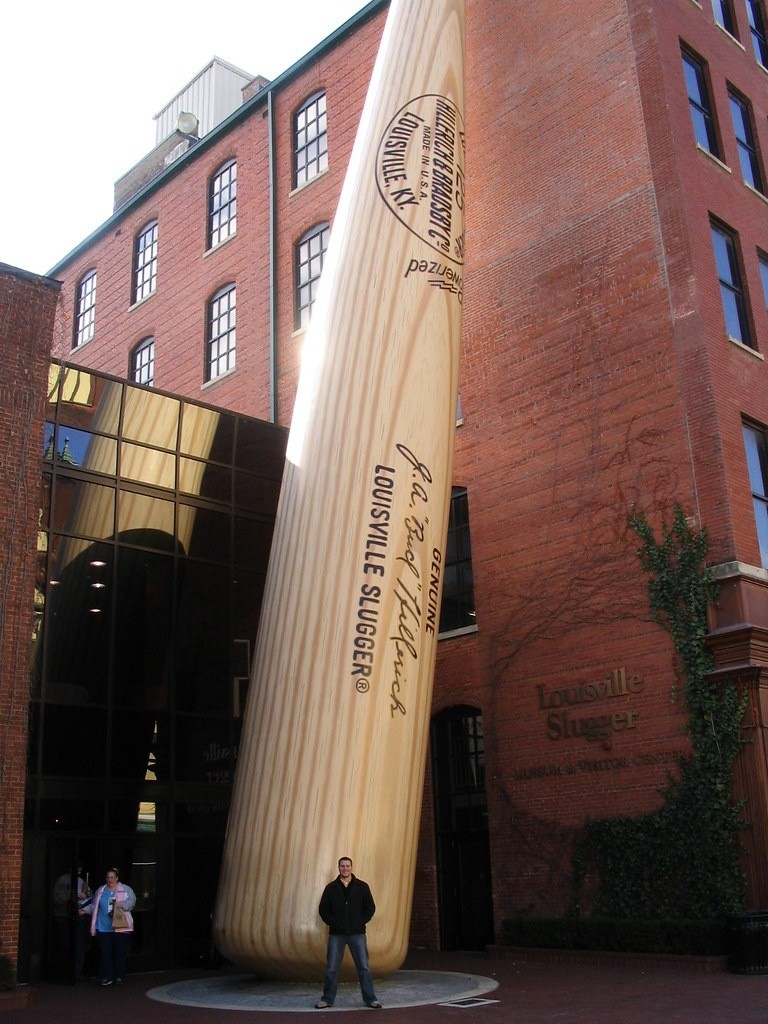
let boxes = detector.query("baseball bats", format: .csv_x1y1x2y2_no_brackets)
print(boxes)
18,378,221,972
207,0,472,977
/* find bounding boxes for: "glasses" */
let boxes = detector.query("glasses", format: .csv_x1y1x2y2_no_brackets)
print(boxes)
106,877,116,880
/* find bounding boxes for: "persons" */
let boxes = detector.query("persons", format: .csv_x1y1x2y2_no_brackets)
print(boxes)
315,857,381,1008
53,864,136,987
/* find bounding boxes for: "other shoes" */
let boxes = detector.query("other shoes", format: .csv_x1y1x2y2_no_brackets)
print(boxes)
115,977,124,984
315,1001,332,1009
368,1000,382,1008
102,979,113,985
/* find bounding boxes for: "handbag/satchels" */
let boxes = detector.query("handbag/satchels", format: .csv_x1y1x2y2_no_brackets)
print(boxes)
112,903,128,928
